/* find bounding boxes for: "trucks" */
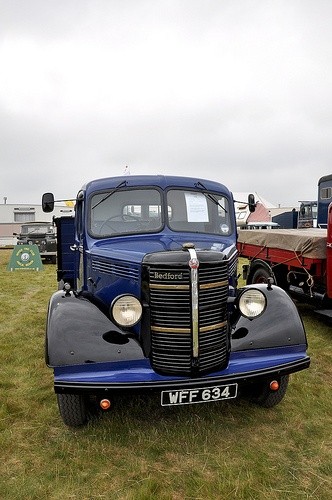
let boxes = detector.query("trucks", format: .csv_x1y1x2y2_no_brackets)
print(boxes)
237,198,332,319
13,223,57,264
39,175,312,428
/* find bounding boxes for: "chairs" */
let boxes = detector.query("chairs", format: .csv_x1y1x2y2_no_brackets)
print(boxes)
93,220,193,235
